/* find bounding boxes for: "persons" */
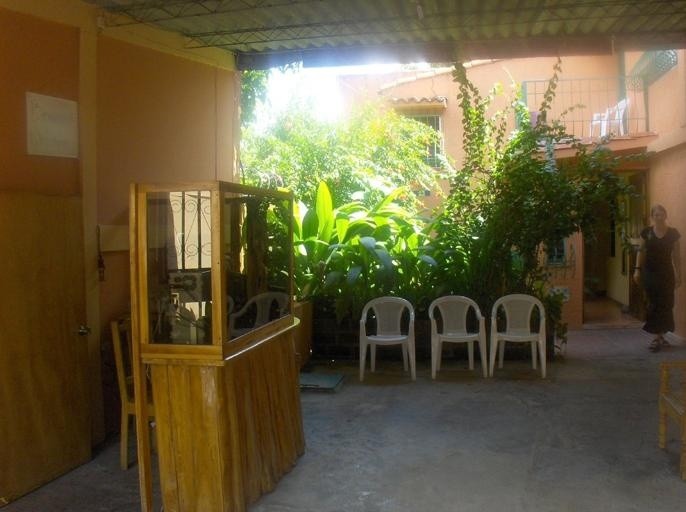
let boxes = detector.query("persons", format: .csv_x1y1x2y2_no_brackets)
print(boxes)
633,205,682,352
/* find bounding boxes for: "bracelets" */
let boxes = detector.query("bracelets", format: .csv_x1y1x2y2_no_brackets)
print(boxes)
634,267,640,271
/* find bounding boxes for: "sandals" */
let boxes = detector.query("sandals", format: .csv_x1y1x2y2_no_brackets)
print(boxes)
659,337,671,348
648,340,660,353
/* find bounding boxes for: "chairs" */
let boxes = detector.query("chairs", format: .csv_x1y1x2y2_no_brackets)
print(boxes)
589,97,630,137
110,315,154,470
430,295,488,380
488,293,547,379
658,358,686,481
229,292,290,337
359,296,417,382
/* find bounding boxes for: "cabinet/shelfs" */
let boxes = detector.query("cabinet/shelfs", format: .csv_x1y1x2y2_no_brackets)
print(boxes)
138,180,294,359
141,316,307,512
